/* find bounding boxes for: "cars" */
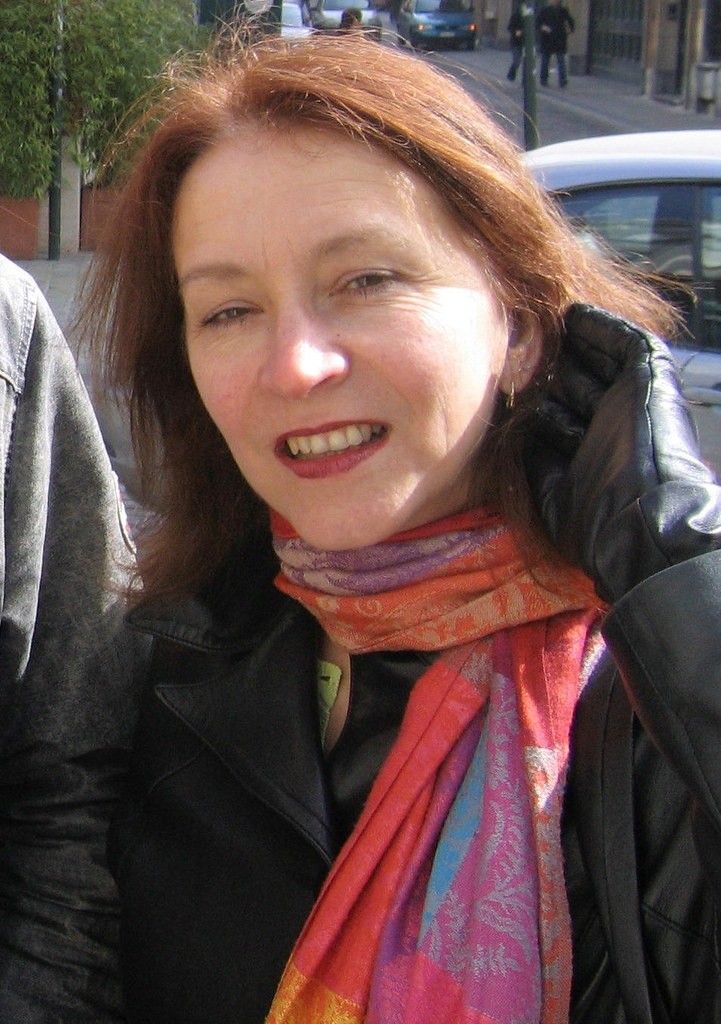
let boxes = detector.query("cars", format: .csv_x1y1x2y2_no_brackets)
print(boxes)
281,0,382,41
520,129,721,483
398,0,479,50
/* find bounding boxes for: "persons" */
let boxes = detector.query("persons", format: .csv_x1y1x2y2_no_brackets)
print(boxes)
95,20,721,1024
0,255,149,1024
335,0,575,91
